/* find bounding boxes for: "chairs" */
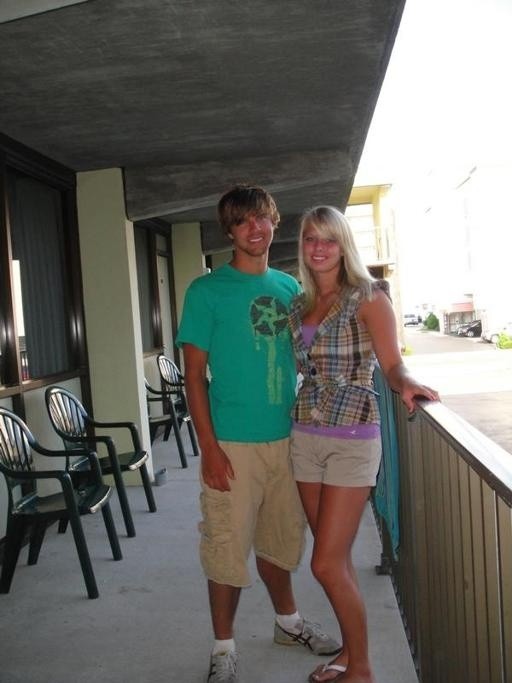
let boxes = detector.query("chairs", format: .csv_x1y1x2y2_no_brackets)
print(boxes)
146,376,199,470
156,354,187,441
45,385,156,536
0,404,122,604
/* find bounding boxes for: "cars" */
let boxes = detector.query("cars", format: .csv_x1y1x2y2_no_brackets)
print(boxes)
482,322,512,343
458,320,481,337
403,314,419,327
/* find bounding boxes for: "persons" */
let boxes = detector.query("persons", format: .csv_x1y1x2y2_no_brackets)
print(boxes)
287,199,442,682
172,185,350,681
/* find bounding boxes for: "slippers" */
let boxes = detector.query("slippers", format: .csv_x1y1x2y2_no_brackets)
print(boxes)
309,660,346,683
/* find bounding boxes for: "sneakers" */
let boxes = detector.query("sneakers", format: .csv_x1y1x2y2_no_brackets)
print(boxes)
208,650,238,683
275,619,342,655
